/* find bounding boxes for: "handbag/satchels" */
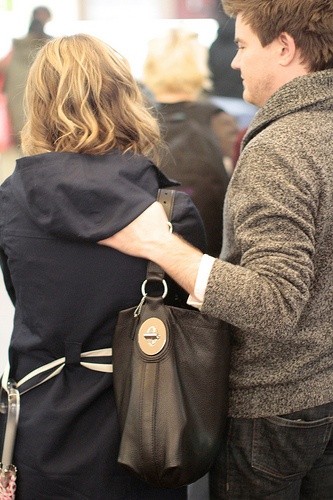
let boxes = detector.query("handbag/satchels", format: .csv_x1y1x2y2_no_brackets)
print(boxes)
110,189,232,487
0,373,21,500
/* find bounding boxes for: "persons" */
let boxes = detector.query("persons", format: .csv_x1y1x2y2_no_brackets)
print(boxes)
0,29,208,500
97,0,333,500
0,5,55,150
